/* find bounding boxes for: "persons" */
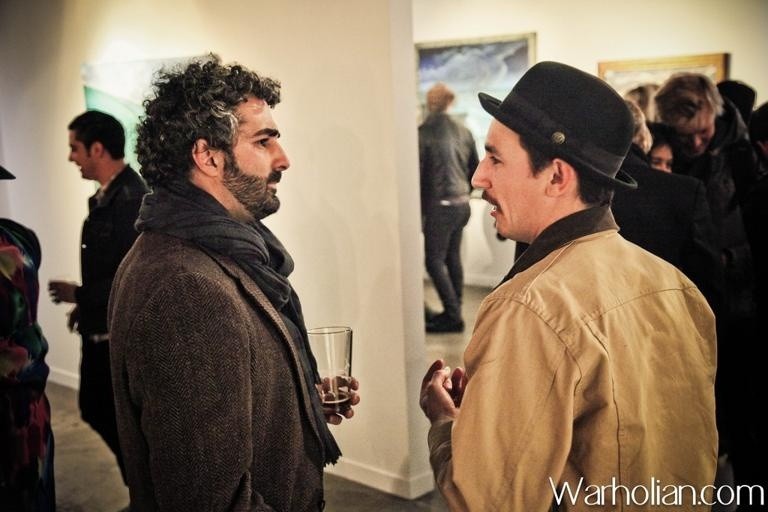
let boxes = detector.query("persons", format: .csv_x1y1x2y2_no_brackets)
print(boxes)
0,162,54,511
417,61,768,512
106,51,361,511
48,111,152,512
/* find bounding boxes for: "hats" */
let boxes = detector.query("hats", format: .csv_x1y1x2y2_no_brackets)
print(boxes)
479,62,639,190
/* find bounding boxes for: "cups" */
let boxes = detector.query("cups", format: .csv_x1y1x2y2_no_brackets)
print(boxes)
306,325,353,412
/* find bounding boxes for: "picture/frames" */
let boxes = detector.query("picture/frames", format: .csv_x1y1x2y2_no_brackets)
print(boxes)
598,54,726,117
414,32,536,199
